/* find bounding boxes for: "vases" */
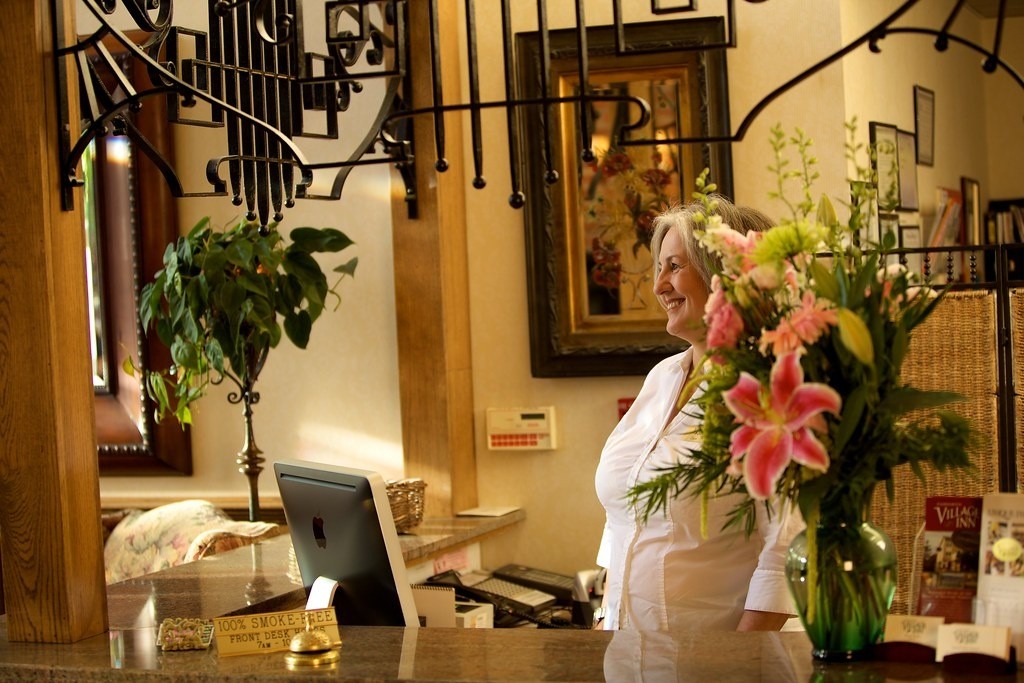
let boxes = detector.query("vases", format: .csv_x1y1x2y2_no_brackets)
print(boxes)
783,488,899,660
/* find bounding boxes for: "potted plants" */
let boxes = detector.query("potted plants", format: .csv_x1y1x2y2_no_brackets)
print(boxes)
111,210,359,521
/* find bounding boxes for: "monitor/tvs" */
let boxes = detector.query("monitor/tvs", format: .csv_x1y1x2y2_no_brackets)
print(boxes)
272,459,421,628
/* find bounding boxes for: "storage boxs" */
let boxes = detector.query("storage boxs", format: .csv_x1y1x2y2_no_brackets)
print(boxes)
456,601,495,630
572,596,602,625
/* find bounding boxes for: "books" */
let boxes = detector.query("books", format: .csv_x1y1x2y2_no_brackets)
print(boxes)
915,495,983,624
925,173,1024,285
409,584,456,628
975,492,1024,635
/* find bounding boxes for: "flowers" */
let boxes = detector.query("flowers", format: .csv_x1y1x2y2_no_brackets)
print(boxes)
620,118,988,649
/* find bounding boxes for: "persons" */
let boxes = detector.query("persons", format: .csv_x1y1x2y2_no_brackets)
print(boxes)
602,631,813,683
593,196,810,631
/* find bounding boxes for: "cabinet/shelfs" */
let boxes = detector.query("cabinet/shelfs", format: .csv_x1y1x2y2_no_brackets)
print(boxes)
984,197,1024,281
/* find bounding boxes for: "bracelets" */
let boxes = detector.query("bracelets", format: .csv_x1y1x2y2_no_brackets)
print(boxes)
593,607,606,621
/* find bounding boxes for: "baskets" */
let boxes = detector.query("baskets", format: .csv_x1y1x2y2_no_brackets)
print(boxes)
385,478,428,533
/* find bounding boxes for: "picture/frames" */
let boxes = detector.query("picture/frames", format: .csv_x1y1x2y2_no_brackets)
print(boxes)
914,84,936,168
850,179,922,284
960,176,980,248
514,15,737,378
896,128,920,212
868,120,901,210
